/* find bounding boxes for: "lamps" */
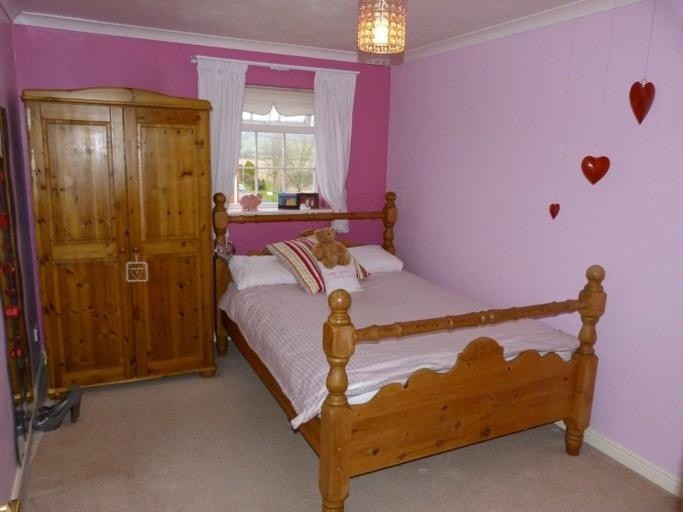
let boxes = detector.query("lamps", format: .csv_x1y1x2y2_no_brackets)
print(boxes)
354,0,407,57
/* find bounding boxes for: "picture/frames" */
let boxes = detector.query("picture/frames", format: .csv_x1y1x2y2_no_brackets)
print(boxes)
296,192,320,211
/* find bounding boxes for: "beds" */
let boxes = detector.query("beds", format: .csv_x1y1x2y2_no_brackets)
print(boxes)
208,188,609,512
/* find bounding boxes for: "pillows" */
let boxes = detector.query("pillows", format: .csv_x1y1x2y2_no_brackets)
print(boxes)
265,234,372,295
315,258,362,297
225,242,406,293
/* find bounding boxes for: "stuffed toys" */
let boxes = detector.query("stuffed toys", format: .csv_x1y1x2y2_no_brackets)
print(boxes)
311,227,353,269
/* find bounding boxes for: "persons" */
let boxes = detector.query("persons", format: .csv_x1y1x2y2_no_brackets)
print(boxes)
301,198,316,208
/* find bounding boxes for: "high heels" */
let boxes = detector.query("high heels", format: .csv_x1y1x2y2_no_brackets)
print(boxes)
32,386,81,431
37,381,82,417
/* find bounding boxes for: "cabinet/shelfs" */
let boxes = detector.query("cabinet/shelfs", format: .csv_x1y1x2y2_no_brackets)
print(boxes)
21,86,219,400
